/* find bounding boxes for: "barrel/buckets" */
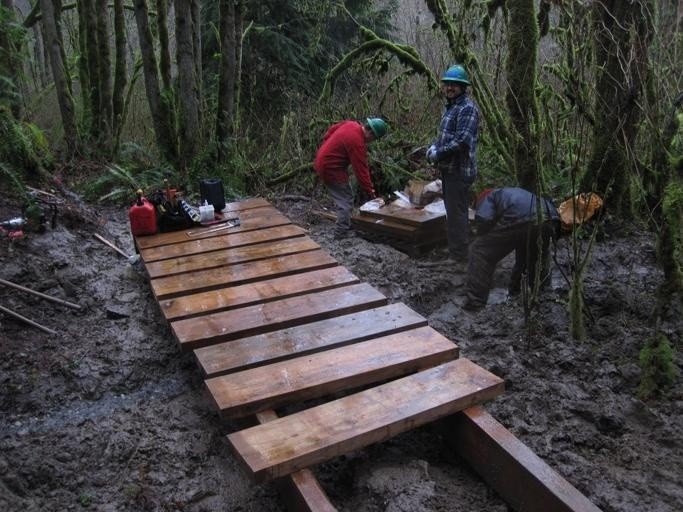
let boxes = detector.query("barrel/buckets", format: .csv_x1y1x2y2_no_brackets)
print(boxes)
129,192,158,236
408,180,437,205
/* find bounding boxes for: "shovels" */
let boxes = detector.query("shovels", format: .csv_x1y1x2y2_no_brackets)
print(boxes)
94,232,140,265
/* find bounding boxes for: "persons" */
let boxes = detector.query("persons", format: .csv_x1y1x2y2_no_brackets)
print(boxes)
425,64,480,264
451,185,559,312
311,116,388,240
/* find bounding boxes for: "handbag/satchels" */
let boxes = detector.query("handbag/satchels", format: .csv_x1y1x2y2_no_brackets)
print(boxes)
159,202,194,232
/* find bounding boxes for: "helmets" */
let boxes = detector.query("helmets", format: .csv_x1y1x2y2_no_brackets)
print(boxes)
367,117,387,138
441,66,470,84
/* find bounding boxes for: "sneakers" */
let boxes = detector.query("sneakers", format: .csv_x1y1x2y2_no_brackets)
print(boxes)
453,297,481,311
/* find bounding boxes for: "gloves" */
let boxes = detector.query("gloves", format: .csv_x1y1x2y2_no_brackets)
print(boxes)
426,144,438,162
